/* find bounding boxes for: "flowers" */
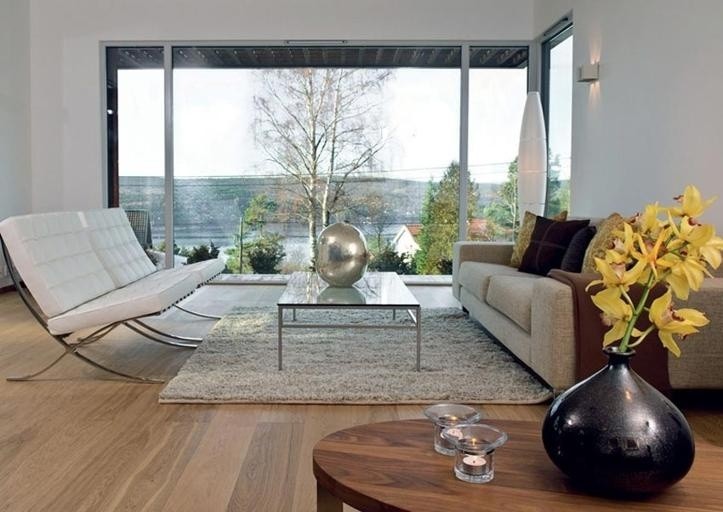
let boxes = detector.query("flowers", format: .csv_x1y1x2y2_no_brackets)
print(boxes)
582,184,723,360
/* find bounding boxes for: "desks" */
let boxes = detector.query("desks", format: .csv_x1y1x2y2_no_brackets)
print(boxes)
309,416,723,512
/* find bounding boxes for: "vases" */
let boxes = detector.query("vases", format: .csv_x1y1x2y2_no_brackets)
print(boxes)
540,346,694,498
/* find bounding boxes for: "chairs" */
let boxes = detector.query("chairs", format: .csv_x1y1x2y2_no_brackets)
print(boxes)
1,205,229,386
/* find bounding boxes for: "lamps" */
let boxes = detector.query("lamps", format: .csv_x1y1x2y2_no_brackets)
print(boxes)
576,63,599,82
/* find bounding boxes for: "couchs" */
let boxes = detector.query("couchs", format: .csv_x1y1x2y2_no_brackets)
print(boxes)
449,239,723,399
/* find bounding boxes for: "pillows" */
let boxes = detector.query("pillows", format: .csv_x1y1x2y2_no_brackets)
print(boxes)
507,210,641,280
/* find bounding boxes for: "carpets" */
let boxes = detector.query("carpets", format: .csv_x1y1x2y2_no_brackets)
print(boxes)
155,305,550,408
